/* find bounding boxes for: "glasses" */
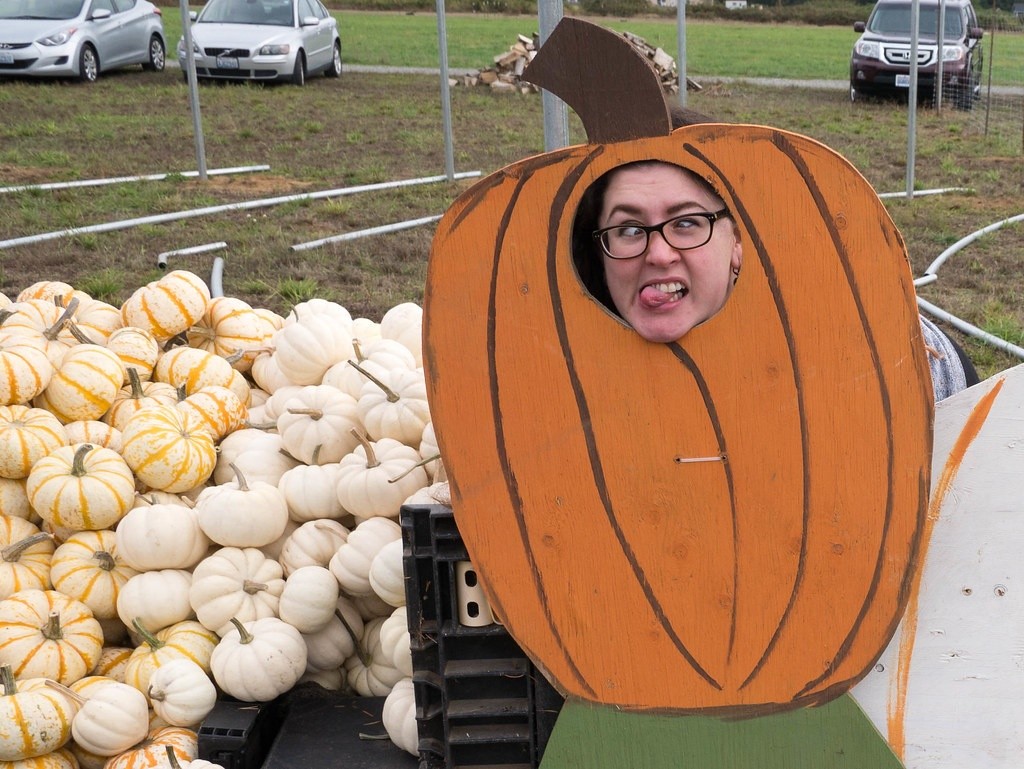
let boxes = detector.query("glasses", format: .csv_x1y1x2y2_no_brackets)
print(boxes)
591,209,729,260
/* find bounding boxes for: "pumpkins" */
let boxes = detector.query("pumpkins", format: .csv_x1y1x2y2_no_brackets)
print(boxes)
0,270,452,769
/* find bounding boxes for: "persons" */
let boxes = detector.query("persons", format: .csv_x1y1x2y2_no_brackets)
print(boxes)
575,160,980,402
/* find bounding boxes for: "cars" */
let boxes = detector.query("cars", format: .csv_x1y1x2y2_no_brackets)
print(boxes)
178,0,342,83
0,0,166,82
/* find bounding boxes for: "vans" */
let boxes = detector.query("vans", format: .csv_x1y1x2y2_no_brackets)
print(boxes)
850,0,983,111
